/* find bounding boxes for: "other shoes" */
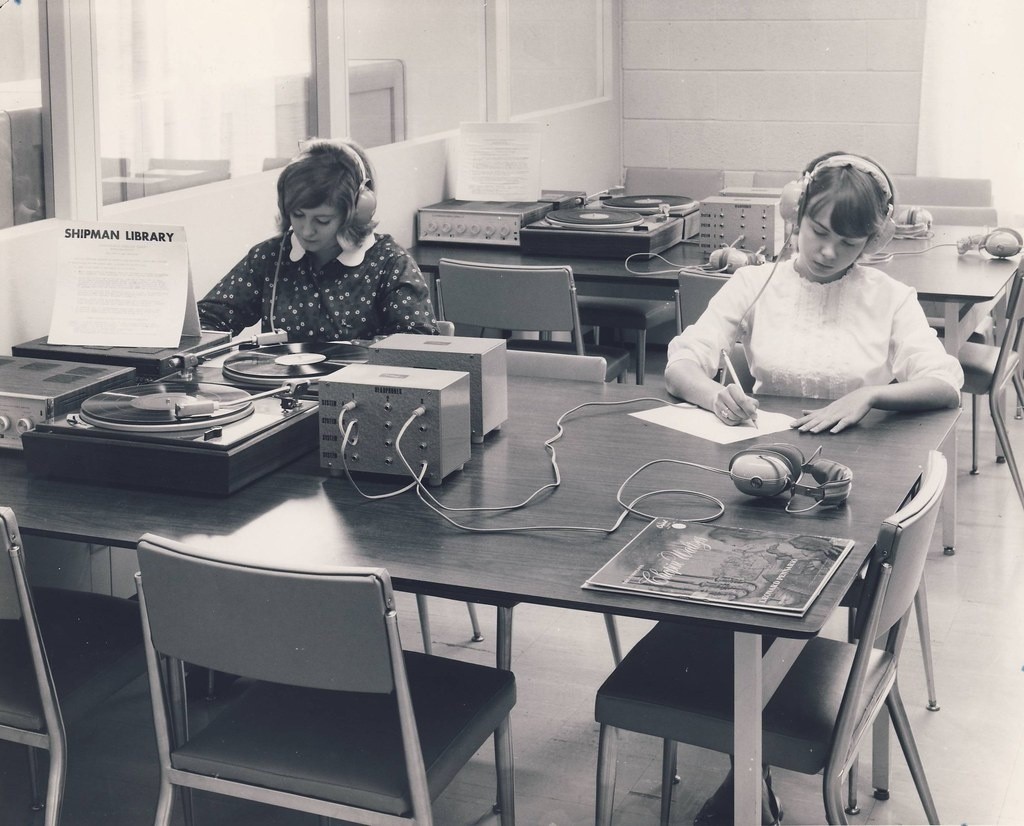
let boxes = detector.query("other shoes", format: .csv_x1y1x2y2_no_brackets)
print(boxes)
693,769,772,826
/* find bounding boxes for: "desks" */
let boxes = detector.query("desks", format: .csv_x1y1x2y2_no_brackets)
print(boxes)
406,225,1023,556
0,375,964,826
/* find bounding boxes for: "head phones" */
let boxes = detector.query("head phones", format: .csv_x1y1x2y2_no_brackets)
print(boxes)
710,246,765,274
894,206,932,238
326,140,376,228
779,156,897,256
956,226,1024,257
728,443,853,507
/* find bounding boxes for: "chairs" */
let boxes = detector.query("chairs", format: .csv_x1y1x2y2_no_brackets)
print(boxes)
594,449,947,826
0,506,517,826
435,167,1024,474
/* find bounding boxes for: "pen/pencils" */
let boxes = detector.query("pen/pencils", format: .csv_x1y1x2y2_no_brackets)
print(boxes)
721,349,759,431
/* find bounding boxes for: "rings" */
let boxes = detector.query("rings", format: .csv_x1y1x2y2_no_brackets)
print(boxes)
721,408,728,418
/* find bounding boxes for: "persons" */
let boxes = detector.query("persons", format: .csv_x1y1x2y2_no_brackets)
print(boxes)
663,152,963,436
196,138,442,341
